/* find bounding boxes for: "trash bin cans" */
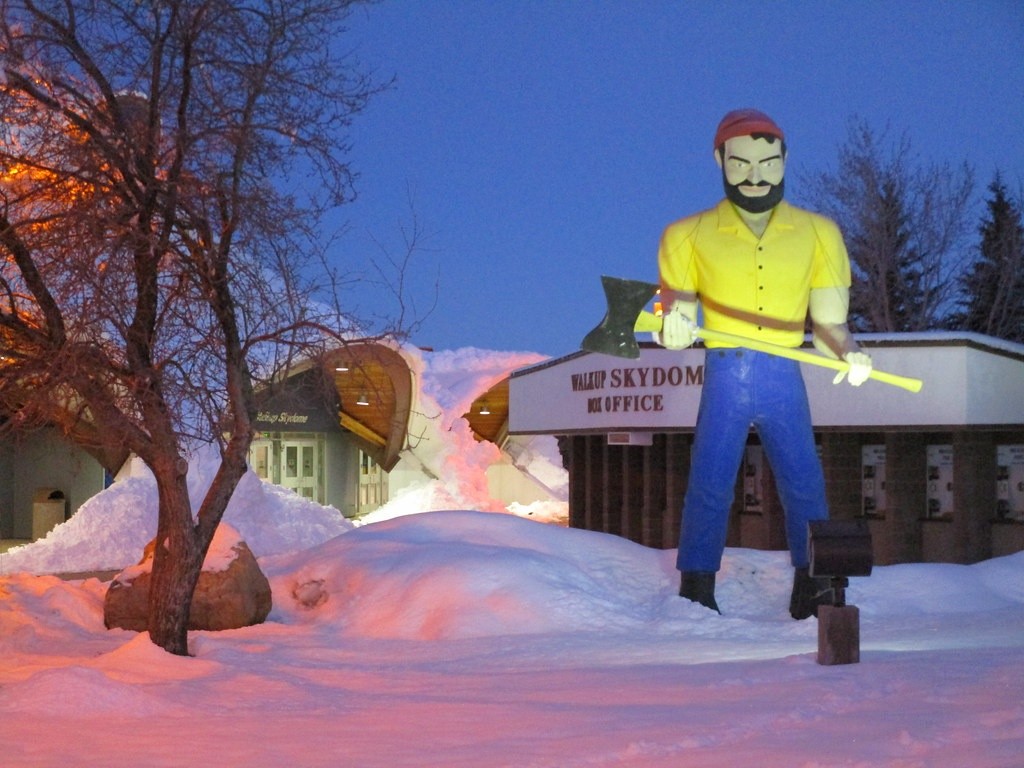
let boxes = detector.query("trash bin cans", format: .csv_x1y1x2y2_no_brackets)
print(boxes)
31,487,67,543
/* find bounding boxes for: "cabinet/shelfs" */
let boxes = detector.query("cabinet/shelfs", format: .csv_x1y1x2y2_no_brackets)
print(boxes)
32,499,66,541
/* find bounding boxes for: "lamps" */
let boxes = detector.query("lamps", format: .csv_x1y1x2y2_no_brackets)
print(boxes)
480,397,491,414
335,360,349,371
356,395,370,406
807,518,874,604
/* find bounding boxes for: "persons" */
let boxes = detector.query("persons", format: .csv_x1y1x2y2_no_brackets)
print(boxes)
652,108,872,620
